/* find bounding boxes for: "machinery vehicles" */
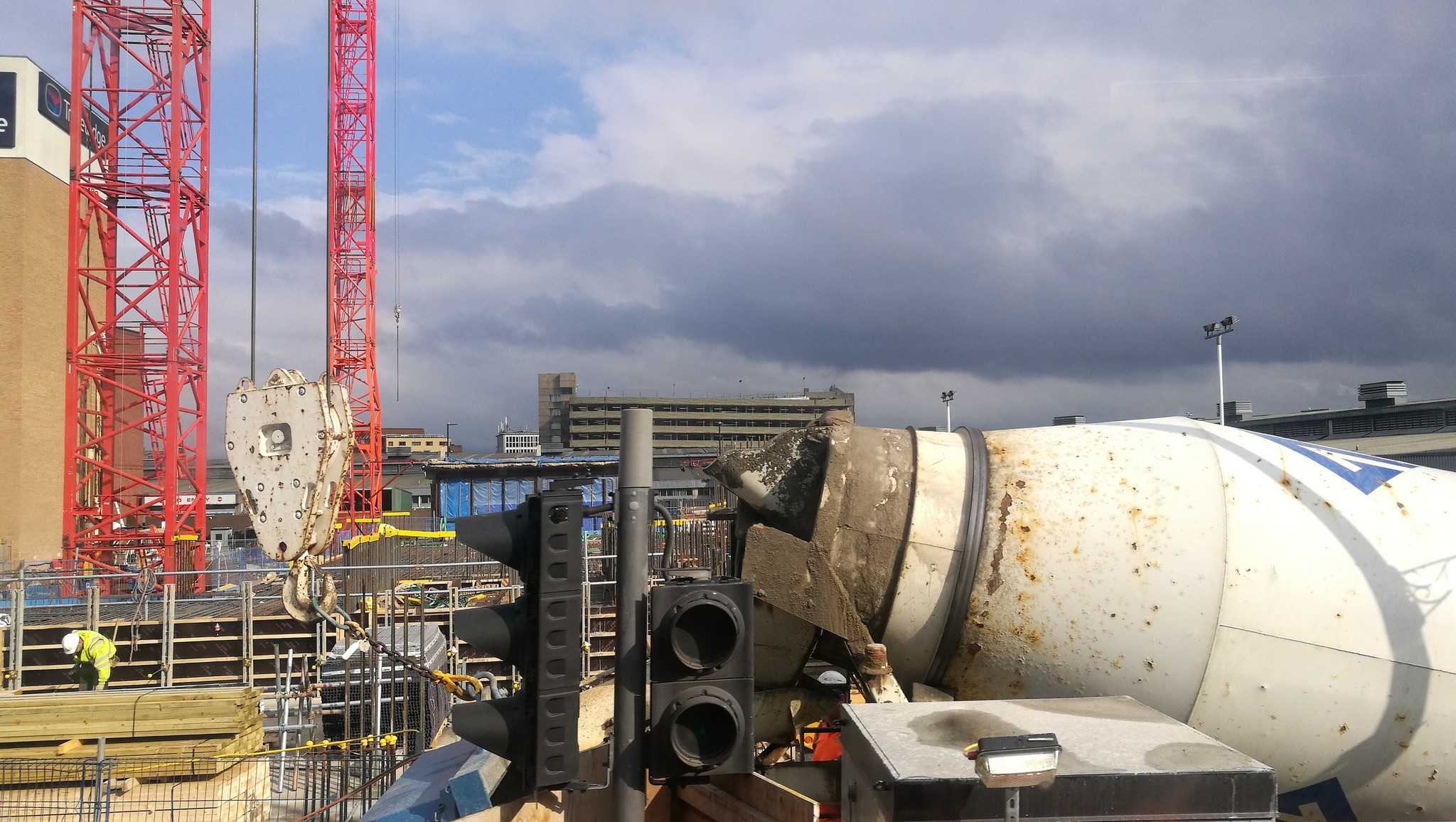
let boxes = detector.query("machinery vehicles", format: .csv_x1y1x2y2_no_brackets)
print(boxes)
695,397,1455,822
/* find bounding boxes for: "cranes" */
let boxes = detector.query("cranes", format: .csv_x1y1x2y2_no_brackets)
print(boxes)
55,1,400,612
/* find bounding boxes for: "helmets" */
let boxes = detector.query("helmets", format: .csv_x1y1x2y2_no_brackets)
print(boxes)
817,669,848,688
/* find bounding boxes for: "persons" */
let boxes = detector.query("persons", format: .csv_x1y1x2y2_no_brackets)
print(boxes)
62,629,116,691
811,669,852,822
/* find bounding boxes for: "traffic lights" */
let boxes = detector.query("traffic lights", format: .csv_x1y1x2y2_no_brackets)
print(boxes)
433,490,585,788
648,583,761,786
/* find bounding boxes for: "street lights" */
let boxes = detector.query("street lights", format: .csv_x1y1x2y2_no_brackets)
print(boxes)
446,423,458,459
1202,313,1241,428
940,390,962,436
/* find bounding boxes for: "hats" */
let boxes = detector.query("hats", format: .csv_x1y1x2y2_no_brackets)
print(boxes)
61,633,80,655
